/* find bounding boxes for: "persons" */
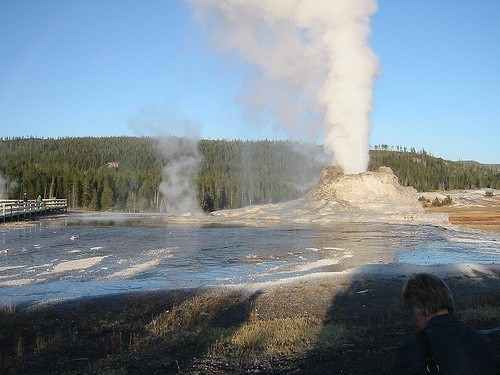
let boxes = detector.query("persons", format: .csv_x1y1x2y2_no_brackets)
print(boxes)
23,192,27,212
392,272,500,375
36,194,41,209
